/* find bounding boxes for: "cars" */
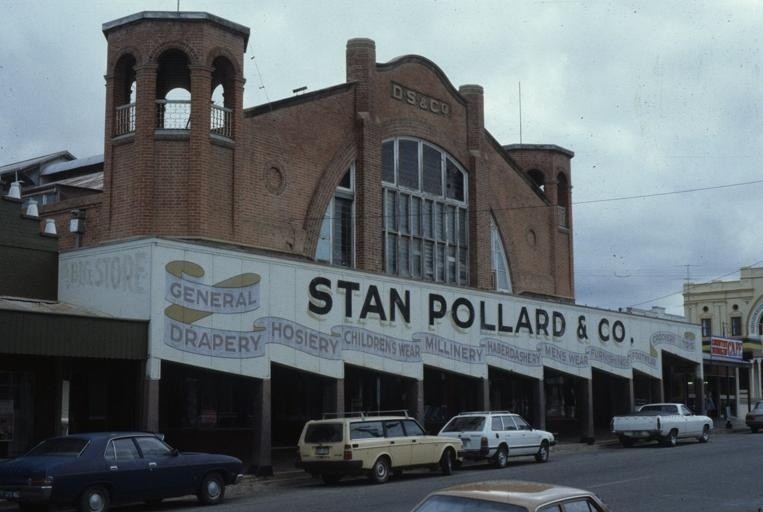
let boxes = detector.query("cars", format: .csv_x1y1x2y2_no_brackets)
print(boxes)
2,433,246,511
406,477,613,512
437,408,561,466
746,401,763,433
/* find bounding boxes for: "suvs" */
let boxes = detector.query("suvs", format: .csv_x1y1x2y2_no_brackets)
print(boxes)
296,409,464,486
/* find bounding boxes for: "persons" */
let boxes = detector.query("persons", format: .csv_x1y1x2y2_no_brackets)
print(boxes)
691,391,728,423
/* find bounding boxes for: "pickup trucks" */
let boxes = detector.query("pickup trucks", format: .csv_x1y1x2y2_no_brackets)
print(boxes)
610,402,715,446
